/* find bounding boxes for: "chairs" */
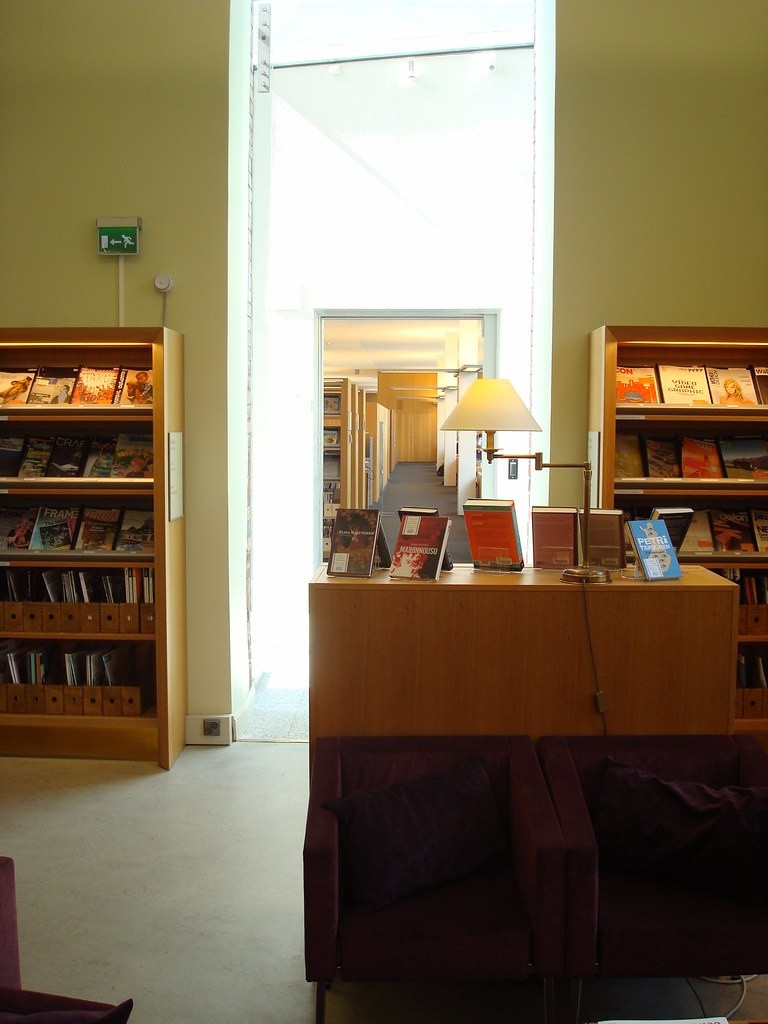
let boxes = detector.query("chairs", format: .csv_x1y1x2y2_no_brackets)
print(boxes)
303,732,768,1024
0,857,135,1024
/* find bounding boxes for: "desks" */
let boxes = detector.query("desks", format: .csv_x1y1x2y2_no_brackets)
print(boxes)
306,564,741,990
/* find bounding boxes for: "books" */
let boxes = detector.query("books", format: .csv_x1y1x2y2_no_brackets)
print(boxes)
737,653,767,688
389,505,454,581
322,397,340,538
722,568,768,606
1,568,154,621
625,520,682,581
648,507,694,557
0,431,154,478
0,504,154,551
531,506,580,570
614,434,768,479
578,506,627,570
616,363,768,406
462,498,526,572
0,366,154,409
614,508,768,555
327,508,392,579
6,647,123,686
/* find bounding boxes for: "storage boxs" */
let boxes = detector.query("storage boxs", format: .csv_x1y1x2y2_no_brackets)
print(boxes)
0,602,155,716
735,605,768,719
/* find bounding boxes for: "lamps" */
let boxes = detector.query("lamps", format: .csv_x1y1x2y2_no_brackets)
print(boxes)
441,379,613,585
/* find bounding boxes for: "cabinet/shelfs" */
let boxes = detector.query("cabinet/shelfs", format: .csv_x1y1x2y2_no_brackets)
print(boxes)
588,325,768,754
323,378,397,558
0,326,189,771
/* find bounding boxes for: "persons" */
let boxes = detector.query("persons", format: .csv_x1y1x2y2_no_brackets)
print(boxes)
124,458,146,478
7,517,32,549
126,380,153,404
719,378,756,406
135,371,153,397
